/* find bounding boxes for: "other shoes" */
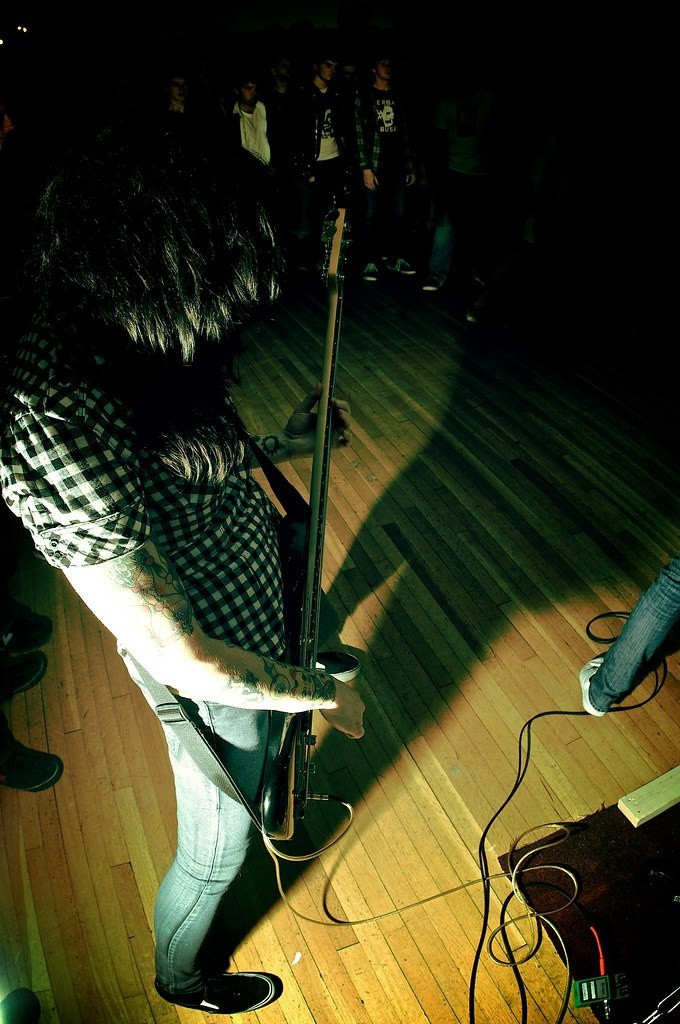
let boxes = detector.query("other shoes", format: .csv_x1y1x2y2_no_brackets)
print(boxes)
386,258,418,275
0,649,49,703
578,656,607,718
0,739,64,794
360,264,380,283
422,272,447,291
0,612,55,648
312,651,361,683
464,305,479,325
153,974,277,1016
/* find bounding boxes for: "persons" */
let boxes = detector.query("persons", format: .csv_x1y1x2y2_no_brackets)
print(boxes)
578,551,680,718
350,51,417,281
143,50,360,281
422,68,493,292
1,132,366,1014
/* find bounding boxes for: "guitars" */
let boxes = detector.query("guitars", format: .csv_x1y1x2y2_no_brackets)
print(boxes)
259,177,352,838
